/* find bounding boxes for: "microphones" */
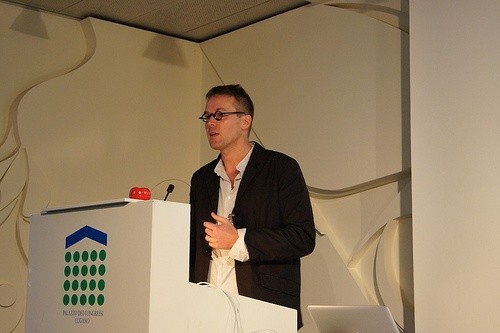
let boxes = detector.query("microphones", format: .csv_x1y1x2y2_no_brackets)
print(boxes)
164,184,174,201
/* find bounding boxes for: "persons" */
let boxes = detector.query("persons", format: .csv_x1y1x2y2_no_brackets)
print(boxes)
187,84,315,330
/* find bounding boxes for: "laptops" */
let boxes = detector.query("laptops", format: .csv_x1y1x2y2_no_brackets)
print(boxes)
308,305,400,333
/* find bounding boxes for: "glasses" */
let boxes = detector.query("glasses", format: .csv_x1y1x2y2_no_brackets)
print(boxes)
198,110,246,122
